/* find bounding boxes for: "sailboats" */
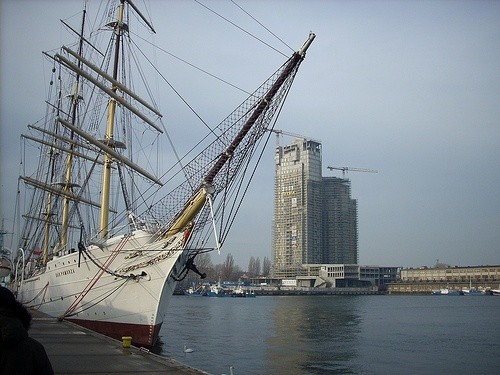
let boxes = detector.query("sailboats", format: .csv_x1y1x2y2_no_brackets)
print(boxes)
0,0,316,345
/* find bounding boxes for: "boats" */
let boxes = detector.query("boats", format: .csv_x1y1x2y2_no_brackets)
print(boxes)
463,289,486,296
182,274,256,298
431,290,464,296
